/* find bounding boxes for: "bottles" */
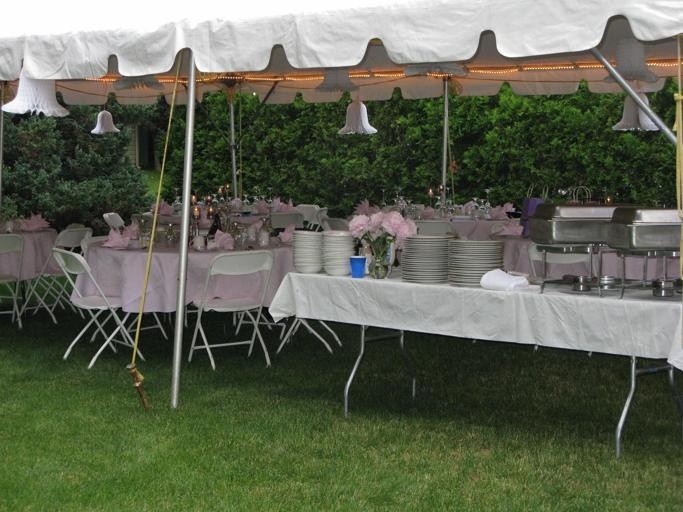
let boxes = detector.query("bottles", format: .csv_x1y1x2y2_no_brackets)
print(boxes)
165,216,270,248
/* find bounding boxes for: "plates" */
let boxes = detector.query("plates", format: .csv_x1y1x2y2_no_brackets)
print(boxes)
402,234,456,283
448,239,506,288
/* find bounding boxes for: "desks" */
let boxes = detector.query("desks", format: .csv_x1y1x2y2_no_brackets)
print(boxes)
267,267,681,457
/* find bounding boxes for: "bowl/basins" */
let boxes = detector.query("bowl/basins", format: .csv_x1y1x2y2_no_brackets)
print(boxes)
292,230,355,275
279,232,293,242
505,211,522,218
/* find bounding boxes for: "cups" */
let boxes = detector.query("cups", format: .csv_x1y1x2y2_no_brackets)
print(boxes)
572,275,591,292
599,275,616,290
652,280,673,297
358,247,372,274
350,256,366,278
137,230,151,249
12,221,21,233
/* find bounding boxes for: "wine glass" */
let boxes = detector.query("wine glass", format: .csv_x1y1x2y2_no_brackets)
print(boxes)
172,187,275,218
391,195,493,221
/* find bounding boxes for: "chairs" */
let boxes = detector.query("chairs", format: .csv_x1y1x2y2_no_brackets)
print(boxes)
0,203,526,372
